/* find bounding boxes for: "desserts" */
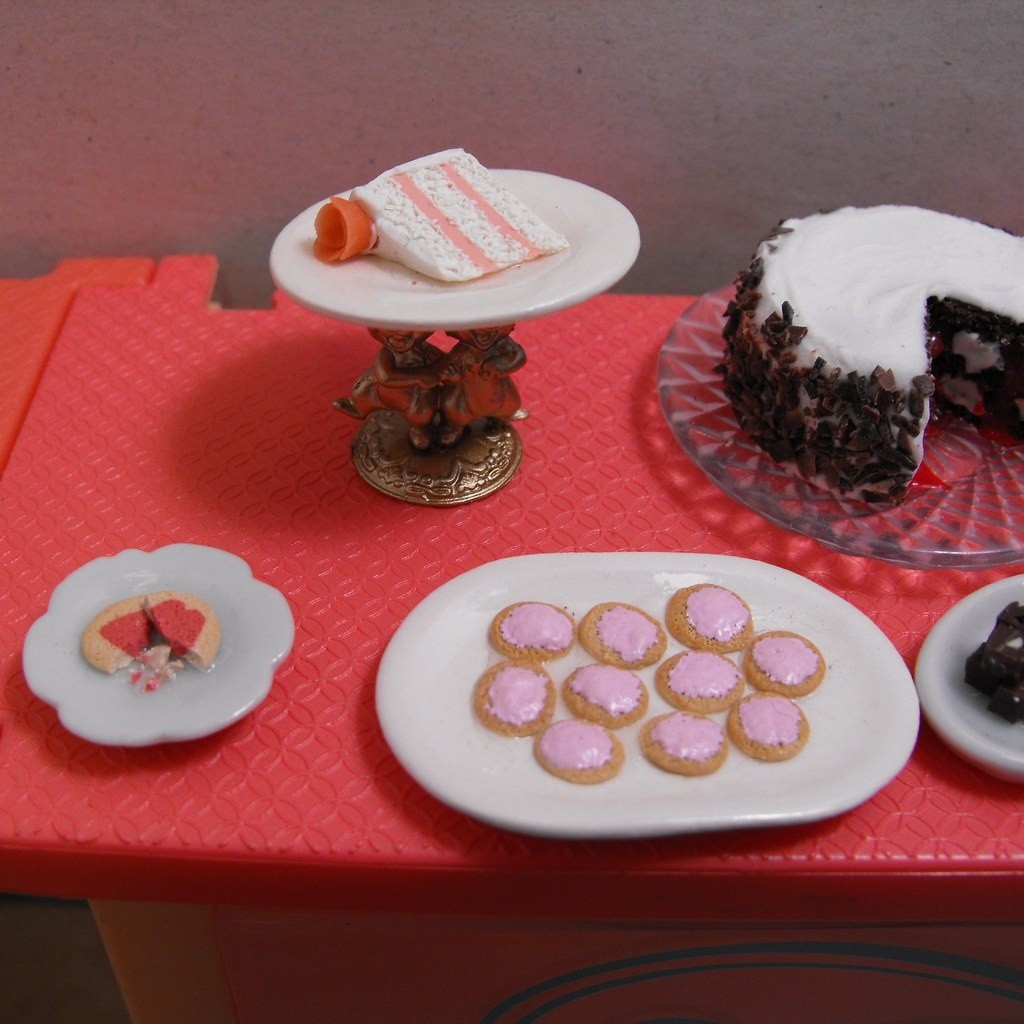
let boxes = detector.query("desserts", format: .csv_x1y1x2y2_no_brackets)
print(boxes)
312,146,571,282
964,600,1024,726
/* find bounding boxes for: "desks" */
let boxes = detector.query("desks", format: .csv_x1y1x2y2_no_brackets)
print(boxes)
0,253,1024,1024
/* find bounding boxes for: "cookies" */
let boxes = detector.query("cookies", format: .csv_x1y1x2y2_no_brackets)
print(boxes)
474,582,829,786
80,590,223,675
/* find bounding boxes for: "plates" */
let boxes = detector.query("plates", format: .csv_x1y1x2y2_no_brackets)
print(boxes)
373,548,922,838
913,570,1024,786
268,166,643,332
21,542,298,748
655,277,1024,571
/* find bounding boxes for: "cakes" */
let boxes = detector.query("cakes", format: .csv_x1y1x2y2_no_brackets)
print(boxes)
719,203,1024,507
